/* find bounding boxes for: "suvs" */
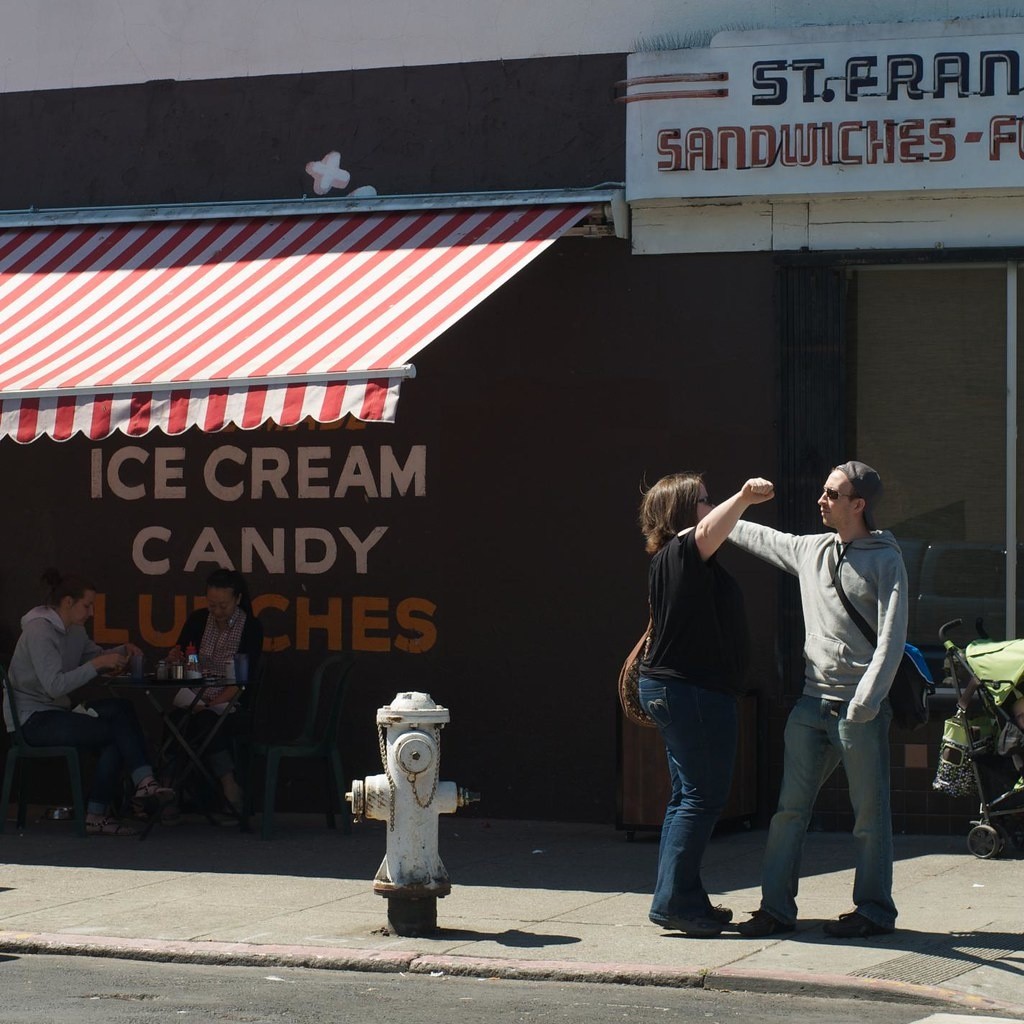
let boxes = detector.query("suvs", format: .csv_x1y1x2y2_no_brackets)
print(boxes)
894,536,1024,643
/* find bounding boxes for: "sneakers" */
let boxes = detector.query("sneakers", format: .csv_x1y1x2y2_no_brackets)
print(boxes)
822,912,895,937
735,909,795,936
220,786,245,827
160,799,185,826
649,903,733,937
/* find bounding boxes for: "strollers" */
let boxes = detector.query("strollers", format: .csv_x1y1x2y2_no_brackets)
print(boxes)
937,617,1024,859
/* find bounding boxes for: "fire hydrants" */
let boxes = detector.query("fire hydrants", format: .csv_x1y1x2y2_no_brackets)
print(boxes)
349,690,478,934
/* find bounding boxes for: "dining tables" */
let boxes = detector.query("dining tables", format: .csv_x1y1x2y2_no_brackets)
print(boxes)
86,672,256,838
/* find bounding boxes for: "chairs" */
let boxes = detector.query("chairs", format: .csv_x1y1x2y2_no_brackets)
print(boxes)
0,663,97,841
237,648,364,835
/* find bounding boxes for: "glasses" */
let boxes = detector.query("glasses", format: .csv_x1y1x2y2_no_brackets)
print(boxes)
823,488,861,500
697,494,712,506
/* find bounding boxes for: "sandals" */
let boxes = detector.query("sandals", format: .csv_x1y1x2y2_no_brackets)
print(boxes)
84,816,140,836
135,779,175,805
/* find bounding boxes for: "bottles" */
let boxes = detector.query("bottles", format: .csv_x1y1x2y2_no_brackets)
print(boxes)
157,660,168,679
186,654,202,679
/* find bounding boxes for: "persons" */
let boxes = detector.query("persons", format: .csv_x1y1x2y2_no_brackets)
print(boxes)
1012,696,1024,731
638,470,776,939
1,565,176,836
726,459,909,938
159,567,264,823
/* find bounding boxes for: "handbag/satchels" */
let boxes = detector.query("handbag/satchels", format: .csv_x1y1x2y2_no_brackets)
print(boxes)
888,643,936,732
932,677,997,797
618,621,661,729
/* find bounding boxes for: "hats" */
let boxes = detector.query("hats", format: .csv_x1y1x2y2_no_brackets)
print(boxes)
837,460,882,531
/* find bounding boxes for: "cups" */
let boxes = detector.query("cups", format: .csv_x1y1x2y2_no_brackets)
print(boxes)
172,665,183,679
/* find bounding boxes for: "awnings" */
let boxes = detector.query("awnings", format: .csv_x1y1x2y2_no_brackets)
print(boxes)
0,202,597,447
